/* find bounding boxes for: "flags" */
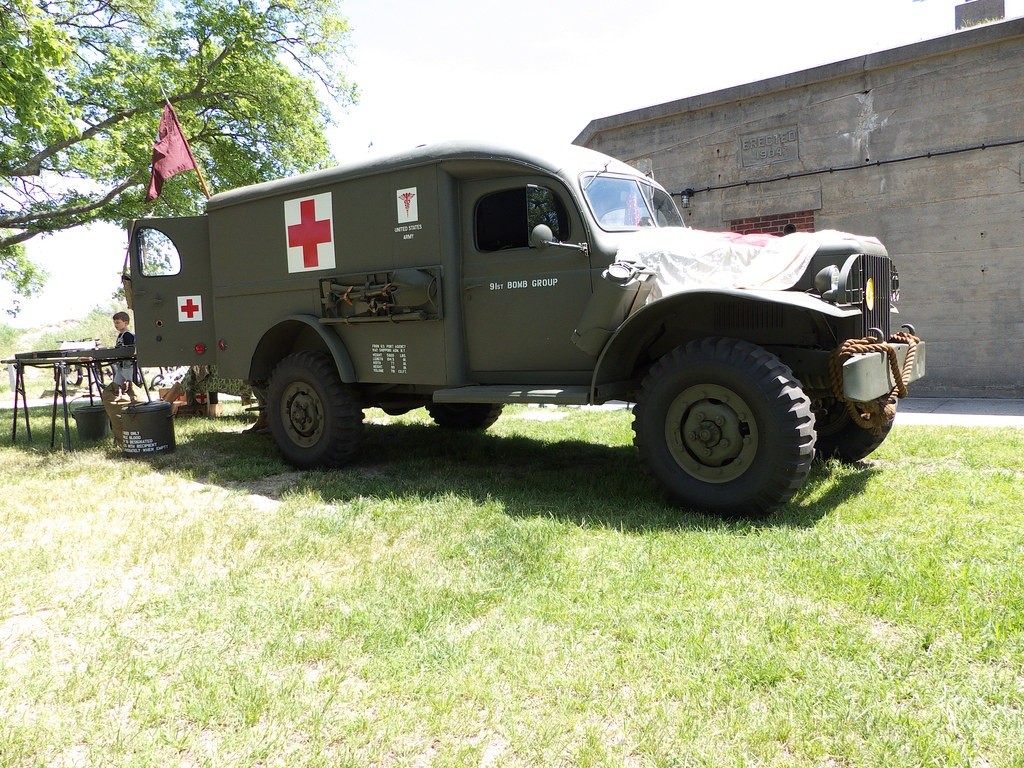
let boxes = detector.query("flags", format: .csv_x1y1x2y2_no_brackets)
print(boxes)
146,105,196,202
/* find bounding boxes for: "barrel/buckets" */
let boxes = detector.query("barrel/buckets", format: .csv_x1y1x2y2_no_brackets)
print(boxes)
68,394,110,442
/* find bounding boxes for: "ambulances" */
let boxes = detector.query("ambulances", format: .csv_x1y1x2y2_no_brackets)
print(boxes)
127,133,928,521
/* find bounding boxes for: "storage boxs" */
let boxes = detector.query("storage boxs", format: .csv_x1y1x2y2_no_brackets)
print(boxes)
159,385,217,418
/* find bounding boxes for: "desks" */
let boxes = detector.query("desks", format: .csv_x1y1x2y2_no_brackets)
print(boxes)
0,353,155,453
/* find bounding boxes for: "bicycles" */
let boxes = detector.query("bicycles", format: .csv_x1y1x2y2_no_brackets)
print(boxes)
54,337,118,390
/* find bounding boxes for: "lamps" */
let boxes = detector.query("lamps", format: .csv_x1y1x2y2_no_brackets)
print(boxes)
680,188,696,209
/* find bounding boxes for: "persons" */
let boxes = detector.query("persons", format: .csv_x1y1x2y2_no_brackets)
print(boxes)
105,312,188,406
